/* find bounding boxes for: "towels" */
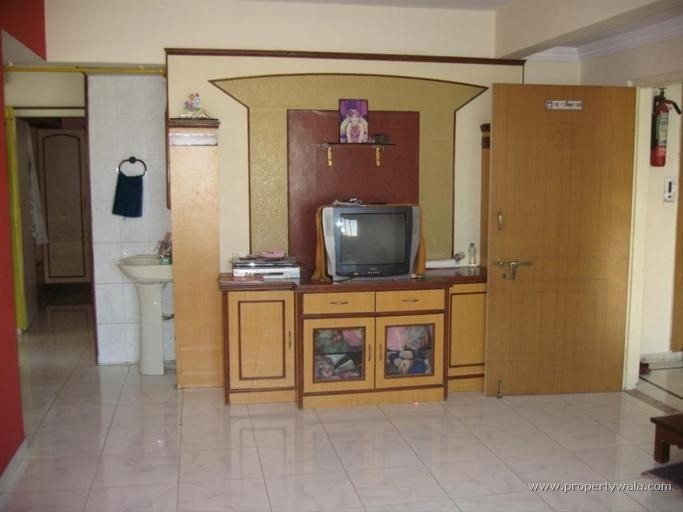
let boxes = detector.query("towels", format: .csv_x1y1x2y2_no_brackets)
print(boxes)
112,171,143,218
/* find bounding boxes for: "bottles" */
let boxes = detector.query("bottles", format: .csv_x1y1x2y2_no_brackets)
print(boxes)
469,243,477,264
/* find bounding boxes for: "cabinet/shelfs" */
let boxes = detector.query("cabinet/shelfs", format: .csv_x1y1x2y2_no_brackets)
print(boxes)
447,279,487,395
296,284,452,409
323,141,396,167
217,272,298,405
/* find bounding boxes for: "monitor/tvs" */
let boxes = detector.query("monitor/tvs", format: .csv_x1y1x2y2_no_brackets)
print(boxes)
320,203,422,281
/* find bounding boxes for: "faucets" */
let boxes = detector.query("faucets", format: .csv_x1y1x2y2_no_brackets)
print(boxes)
154,239,167,256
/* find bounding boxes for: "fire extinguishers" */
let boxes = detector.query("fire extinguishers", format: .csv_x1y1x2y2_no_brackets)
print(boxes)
650,87,681,166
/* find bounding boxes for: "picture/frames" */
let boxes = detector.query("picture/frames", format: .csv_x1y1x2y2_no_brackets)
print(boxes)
338,98,369,143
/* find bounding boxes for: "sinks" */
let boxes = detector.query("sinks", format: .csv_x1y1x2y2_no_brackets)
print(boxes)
117,254,172,284
